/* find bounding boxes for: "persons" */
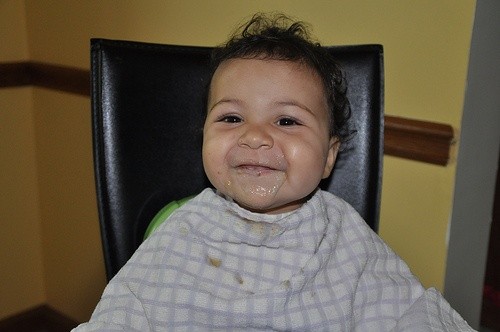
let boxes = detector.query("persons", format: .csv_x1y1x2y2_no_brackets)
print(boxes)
110,10,440,331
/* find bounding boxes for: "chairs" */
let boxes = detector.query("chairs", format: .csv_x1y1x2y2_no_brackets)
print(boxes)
89,37,385,282
